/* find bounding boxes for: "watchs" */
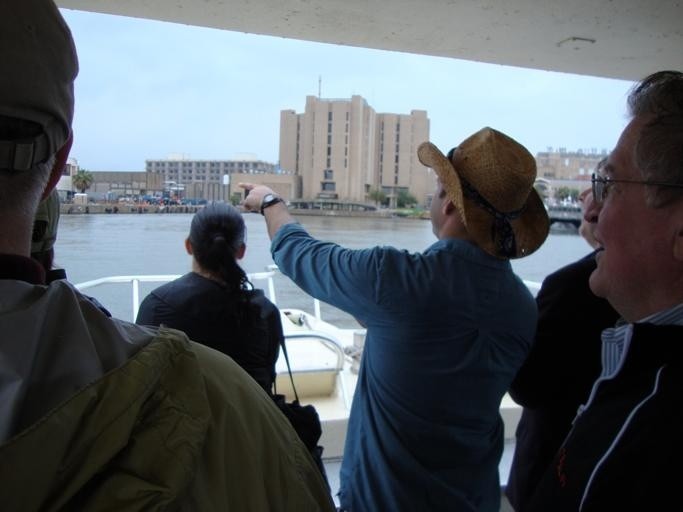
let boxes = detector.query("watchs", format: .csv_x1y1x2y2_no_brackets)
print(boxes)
260,193,286,216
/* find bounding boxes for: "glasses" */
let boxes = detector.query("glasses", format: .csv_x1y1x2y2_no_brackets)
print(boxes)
592,173,669,204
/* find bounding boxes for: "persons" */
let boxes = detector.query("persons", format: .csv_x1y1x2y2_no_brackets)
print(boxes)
527,70,683,511
236,127,538,510
134,201,281,395
506,150,615,510
0,0,337,510
31,187,112,317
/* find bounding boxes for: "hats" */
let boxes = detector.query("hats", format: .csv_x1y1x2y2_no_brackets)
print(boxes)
416,127,550,260
0,0,79,172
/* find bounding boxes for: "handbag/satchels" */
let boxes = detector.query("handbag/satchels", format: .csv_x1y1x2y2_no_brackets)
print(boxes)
270,392,332,493
